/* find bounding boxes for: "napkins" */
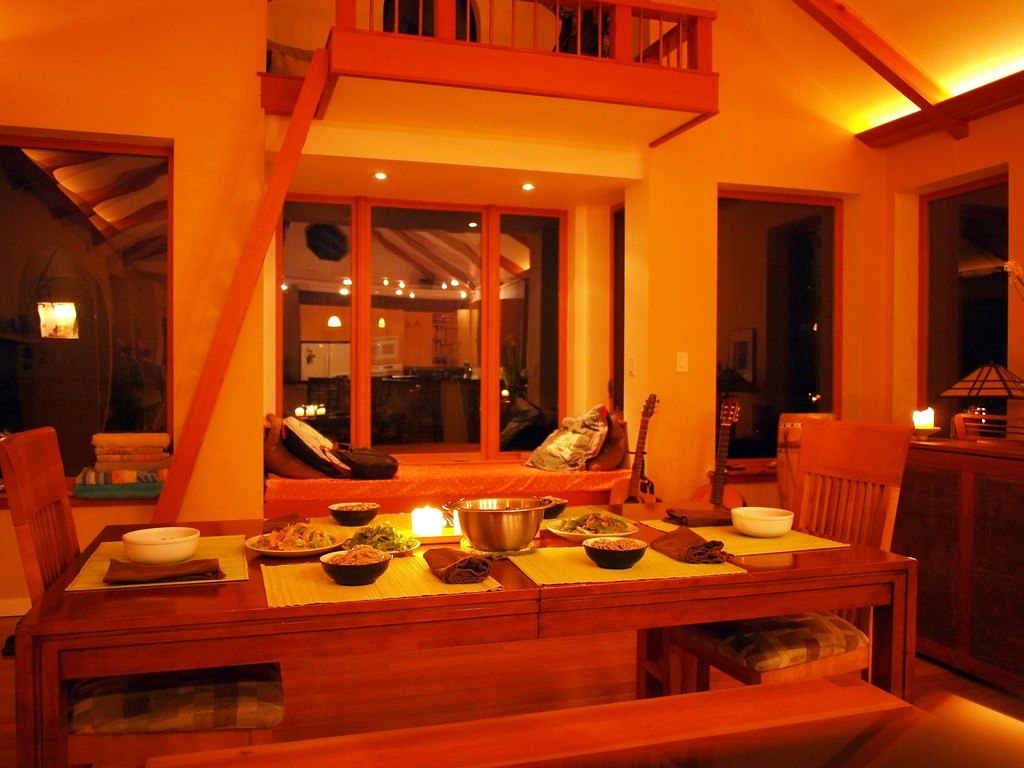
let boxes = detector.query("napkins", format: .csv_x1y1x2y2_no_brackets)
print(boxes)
263,512,309,535
663,507,732,526
423,546,493,585
649,526,730,565
103,558,226,584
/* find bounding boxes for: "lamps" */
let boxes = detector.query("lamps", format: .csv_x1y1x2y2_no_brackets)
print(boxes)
936,363,1024,437
38,302,78,339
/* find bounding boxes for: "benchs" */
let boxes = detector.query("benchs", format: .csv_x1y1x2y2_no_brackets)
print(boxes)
144,669,918,768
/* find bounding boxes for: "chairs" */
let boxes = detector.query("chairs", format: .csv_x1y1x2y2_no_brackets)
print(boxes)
306,375,407,444
665,418,915,694
0,427,286,768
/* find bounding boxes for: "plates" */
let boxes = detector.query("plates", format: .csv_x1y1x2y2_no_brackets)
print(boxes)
245,529,347,557
342,538,421,557
546,518,639,542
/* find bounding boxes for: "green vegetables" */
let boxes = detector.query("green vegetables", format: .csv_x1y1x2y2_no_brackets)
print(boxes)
344,521,416,552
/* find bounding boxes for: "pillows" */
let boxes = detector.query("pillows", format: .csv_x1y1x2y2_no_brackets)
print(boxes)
526,404,627,472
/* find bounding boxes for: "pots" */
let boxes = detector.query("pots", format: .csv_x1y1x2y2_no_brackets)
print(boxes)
441,497,556,551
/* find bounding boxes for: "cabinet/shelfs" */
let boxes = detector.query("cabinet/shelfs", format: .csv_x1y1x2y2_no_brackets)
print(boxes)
891,440,1024,701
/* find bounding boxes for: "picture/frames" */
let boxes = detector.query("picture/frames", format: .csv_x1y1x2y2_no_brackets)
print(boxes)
727,327,755,385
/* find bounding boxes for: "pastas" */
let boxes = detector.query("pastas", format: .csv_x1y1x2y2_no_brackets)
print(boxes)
589,539,641,550
327,545,389,565
336,502,377,511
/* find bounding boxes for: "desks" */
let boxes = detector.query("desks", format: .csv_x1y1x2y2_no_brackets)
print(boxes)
15,501,918,768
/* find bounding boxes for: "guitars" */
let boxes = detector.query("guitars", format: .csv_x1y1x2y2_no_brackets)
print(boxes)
608,392,662,504
689,396,748,508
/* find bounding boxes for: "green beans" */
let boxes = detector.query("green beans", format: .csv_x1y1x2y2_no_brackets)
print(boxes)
558,513,630,534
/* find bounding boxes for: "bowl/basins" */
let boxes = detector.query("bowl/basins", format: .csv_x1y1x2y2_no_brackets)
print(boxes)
582,536,648,570
320,549,393,586
543,499,568,519
122,526,201,565
731,506,795,539
327,503,380,526
